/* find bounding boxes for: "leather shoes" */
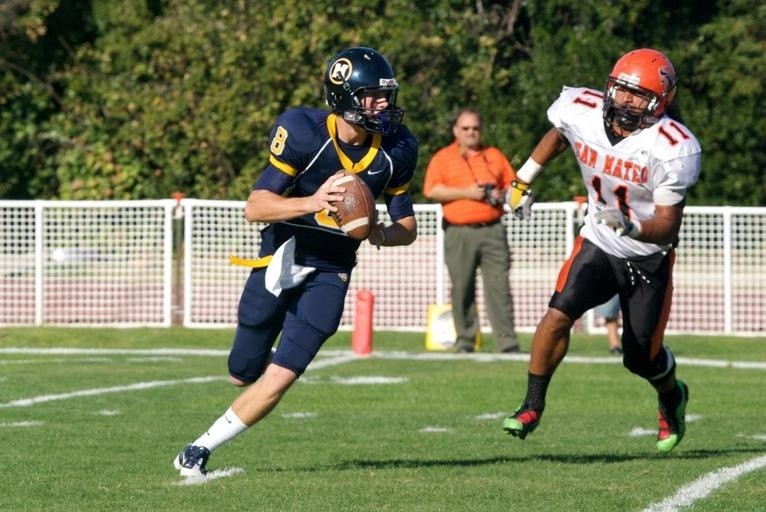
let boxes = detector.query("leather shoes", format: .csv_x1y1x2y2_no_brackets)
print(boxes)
457,347,474,353
503,346,519,352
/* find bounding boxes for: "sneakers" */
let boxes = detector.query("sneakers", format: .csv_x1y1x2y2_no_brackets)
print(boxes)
173,441,211,477
656,380,689,453
503,401,539,440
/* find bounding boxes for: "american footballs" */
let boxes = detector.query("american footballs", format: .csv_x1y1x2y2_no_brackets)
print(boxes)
332,170,376,240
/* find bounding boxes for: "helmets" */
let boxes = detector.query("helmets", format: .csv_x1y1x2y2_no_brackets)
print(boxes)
602,48,679,132
323,47,405,136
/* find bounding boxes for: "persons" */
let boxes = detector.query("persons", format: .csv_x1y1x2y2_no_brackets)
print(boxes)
502,48,701,452
604,295,627,355
425,108,520,353
174,48,417,476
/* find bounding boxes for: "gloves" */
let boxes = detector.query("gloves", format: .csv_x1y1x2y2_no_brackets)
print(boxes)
505,157,545,220
593,201,641,239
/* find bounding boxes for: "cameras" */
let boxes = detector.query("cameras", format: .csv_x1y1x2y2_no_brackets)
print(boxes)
478,183,500,207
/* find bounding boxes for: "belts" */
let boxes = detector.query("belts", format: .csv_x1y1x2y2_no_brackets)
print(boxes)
467,218,501,229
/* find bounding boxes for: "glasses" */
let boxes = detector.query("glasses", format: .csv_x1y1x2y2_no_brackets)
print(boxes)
462,126,480,131
